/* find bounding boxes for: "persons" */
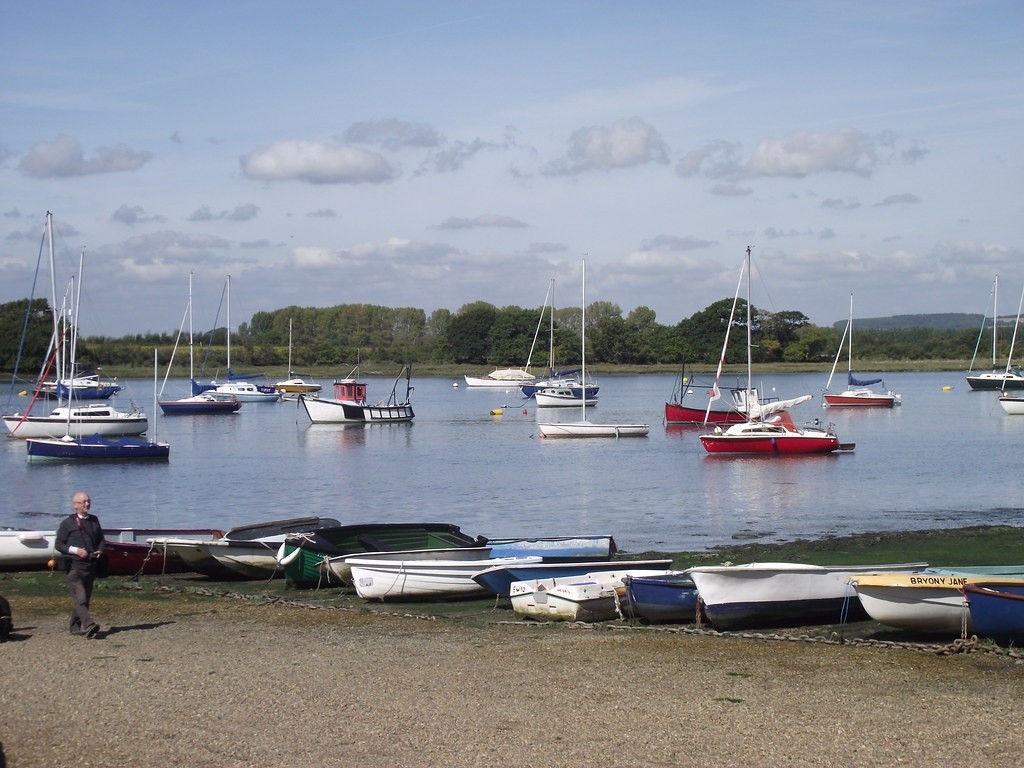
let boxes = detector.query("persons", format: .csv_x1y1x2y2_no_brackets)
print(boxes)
54,493,106,638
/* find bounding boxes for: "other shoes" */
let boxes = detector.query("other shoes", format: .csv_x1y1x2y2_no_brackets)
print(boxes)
86,624,100,638
71,630,86,636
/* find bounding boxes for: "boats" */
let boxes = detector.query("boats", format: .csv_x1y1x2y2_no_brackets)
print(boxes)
662,353,780,424
484,533,618,563
961,580,1023,650
324,534,493,590
157,392,242,415
146,516,489,588
344,555,544,601
684,561,930,634
200,381,281,402
462,374,534,386
0,529,63,569
98,528,227,573
534,388,598,408
510,569,691,621
300,347,415,424
842,570,986,634
922,564,1024,579
471,559,674,600
621,573,708,625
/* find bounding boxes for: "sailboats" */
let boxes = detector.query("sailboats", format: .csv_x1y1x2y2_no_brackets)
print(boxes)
697,245,856,454
965,274,1024,390
519,279,601,400
30,275,123,401
823,292,903,407
270,318,324,394
192,273,276,396
1,210,148,439
996,288,1024,416
536,258,650,438
26,252,171,461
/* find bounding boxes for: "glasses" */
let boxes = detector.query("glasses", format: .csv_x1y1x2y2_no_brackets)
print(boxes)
75,500,91,504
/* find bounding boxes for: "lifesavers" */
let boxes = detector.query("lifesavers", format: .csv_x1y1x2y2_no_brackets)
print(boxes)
275,542,303,567
